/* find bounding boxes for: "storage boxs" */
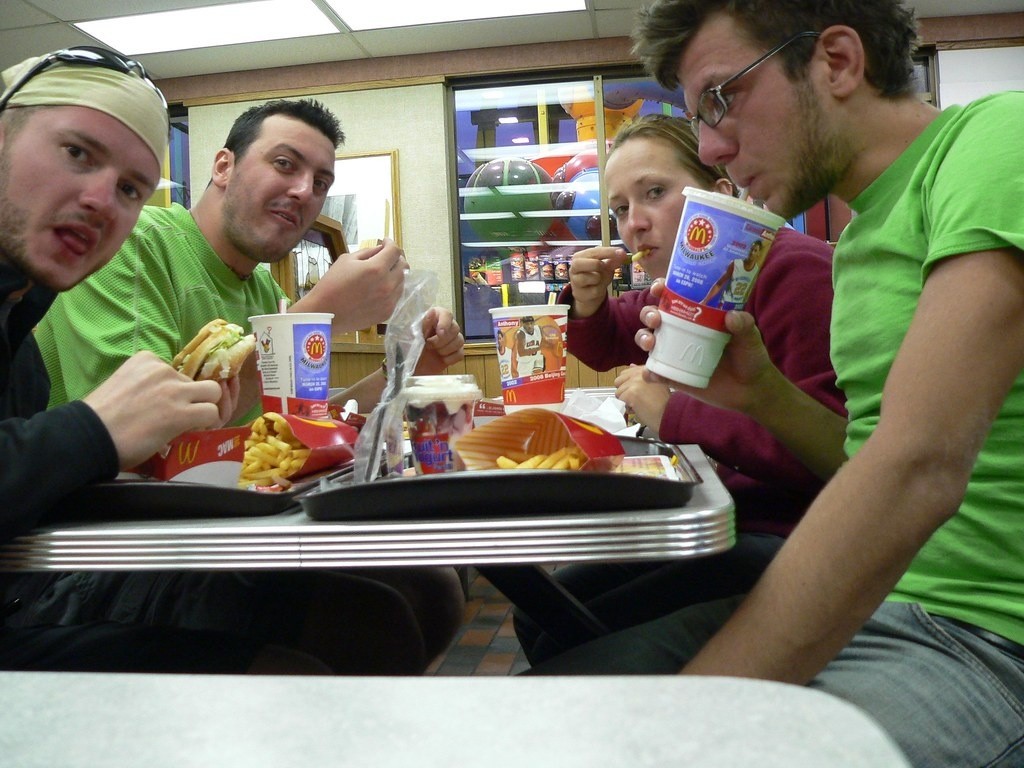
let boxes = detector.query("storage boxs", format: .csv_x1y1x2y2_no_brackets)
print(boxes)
146,427,253,482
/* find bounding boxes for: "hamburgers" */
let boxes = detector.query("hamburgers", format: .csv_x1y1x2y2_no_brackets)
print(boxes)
172,318,258,385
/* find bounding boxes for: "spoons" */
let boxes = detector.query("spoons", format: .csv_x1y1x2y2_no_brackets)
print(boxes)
362,343,405,482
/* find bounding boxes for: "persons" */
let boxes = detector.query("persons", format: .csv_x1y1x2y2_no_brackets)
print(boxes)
507,113,847,678
0,44,330,673
33,96,468,675
508,0,1024,765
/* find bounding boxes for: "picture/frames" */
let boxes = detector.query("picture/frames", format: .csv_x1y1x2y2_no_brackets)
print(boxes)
270,148,401,308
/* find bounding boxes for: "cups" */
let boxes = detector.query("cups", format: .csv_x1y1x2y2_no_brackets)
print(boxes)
646,183,786,392
248,312,335,420
488,304,571,413
400,374,482,477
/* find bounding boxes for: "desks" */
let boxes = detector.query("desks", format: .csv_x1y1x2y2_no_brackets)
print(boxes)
0,671,918,768
0,442,736,646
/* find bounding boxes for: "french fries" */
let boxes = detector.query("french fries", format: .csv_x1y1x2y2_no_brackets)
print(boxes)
238,412,310,488
494,446,587,470
632,249,650,261
384,199,391,238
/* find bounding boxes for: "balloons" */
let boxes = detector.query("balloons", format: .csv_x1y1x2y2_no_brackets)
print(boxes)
462,156,557,261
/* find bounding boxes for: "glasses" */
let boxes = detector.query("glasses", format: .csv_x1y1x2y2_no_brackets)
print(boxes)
0,44,170,113
690,30,820,141
